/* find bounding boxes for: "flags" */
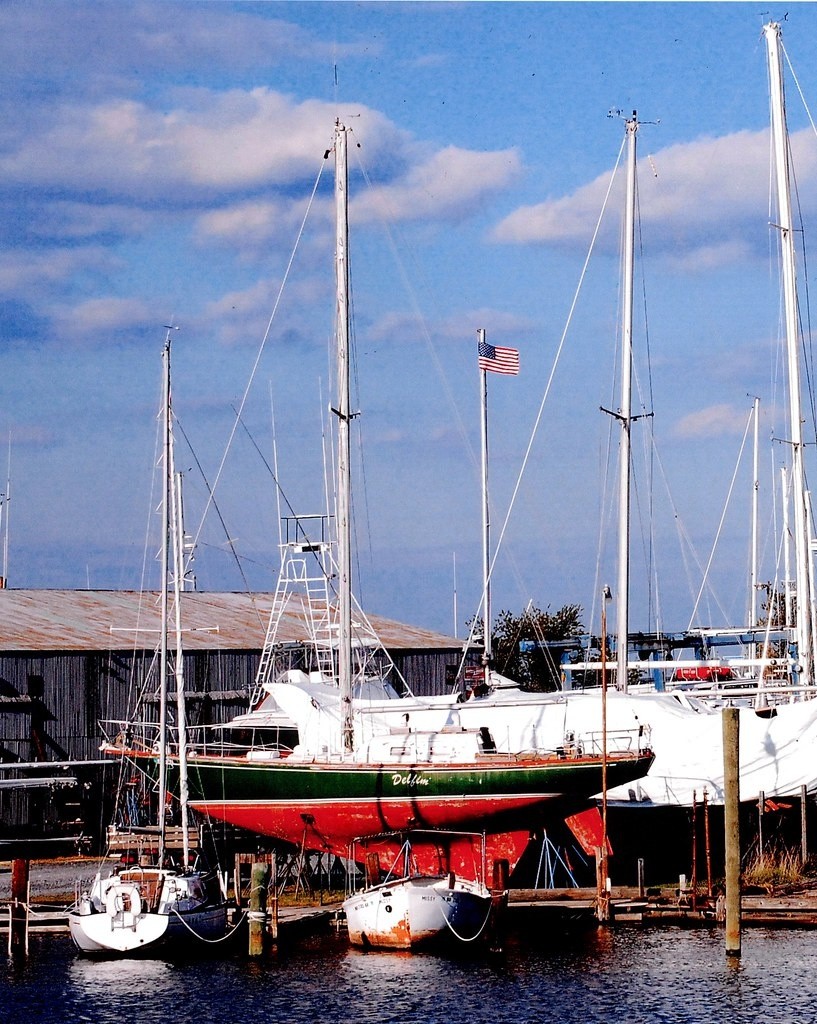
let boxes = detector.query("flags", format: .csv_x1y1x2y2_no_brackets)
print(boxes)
477,342,519,376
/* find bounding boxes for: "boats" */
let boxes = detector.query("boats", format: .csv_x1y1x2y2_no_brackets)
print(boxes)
335,871,492,950
69,873,229,954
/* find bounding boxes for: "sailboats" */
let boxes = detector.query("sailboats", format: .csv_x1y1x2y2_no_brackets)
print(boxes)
208,110,817,813
517,10,817,712
96,62,656,891
